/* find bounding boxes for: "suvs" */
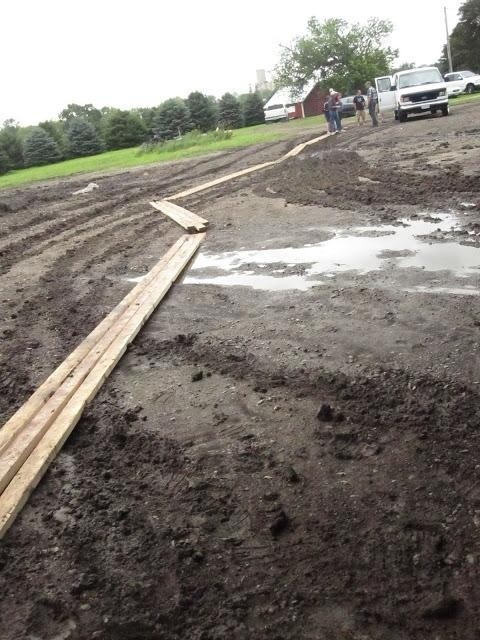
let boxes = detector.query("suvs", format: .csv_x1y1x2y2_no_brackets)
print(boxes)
338,65,480,123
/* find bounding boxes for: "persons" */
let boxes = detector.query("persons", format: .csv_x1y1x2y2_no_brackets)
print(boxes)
352,89,366,126
365,80,379,127
322,87,348,135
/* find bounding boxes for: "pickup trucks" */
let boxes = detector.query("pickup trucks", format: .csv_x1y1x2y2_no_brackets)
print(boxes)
262,103,289,122
